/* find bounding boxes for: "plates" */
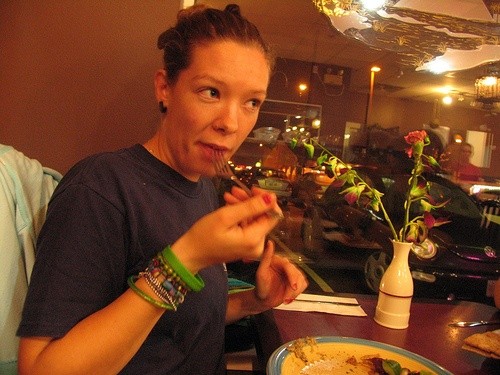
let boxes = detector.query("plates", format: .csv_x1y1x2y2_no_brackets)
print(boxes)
266,335,452,375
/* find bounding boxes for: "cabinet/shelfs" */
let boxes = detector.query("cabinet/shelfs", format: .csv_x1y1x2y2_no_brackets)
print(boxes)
244,100,323,144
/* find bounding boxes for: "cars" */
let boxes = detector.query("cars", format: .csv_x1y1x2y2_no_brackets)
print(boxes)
241,166,500,297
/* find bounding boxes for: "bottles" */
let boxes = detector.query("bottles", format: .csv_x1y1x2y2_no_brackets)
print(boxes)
279,199,291,238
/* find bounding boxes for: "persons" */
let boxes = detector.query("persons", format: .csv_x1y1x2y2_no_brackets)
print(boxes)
16,5,308,374
444,143,481,181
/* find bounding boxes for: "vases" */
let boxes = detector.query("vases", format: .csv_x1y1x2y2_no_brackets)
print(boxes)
374,240,413,330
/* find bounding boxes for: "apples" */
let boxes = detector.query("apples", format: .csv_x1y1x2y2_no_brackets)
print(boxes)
382,359,401,375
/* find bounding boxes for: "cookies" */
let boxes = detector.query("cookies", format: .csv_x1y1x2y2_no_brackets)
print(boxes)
464,332,500,355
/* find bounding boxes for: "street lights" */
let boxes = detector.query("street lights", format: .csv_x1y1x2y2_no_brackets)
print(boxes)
363,66,381,150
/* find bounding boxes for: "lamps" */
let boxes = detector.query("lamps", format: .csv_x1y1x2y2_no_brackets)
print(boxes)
324,68,344,86
474,62,500,110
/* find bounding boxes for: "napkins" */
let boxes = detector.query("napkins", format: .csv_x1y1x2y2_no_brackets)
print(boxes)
273,293,367,317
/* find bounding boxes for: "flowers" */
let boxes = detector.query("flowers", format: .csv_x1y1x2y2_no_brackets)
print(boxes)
289,130,451,251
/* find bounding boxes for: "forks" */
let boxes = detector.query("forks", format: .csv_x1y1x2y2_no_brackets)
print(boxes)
210,150,254,199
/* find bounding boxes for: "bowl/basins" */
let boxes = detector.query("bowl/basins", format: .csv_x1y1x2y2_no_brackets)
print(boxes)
252,126,280,141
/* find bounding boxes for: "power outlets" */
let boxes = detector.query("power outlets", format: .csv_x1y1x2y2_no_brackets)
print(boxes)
312,66,318,73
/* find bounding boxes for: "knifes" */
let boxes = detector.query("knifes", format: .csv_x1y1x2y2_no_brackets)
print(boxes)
451,320,500,327
294,299,362,306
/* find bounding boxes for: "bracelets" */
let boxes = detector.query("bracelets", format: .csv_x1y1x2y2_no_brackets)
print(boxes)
127,245,204,310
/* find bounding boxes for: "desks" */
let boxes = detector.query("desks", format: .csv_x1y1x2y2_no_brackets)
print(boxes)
273,201,375,269
251,292,500,375
396,223,500,303
